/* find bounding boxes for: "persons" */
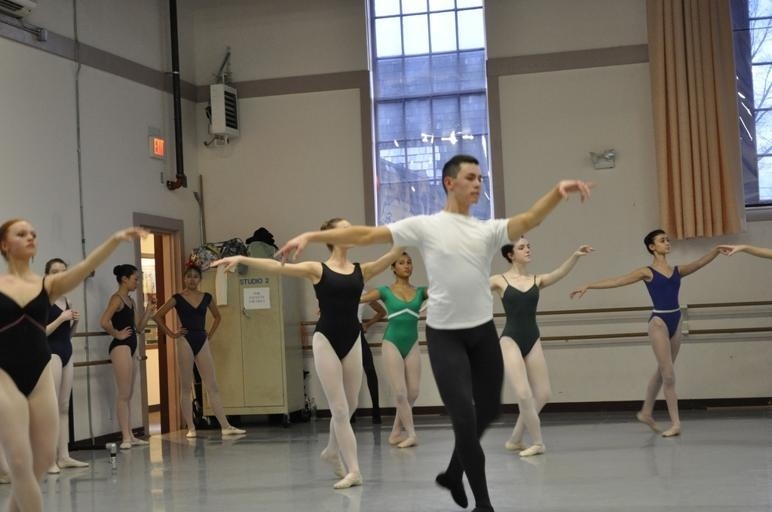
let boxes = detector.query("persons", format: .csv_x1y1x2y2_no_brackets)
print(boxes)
719,242,772,263
313,250,430,450
349,288,388,426
212,216,407,491
152,263,246,440
99,263,152,451
41,258,90,474
570,228,723,440
270,152,598,512
486,231,596,459
0,217,152,512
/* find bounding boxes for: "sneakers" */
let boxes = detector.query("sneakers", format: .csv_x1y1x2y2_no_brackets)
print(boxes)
389,436,416,450
120,439,150,449
48,457,89,473
636,412,680,438
186,432,196,438
322,450,363,490
505,442,545,456
222,427,246,434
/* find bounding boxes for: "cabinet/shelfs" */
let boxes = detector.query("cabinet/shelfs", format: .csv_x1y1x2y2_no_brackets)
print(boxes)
196,263,308,425
